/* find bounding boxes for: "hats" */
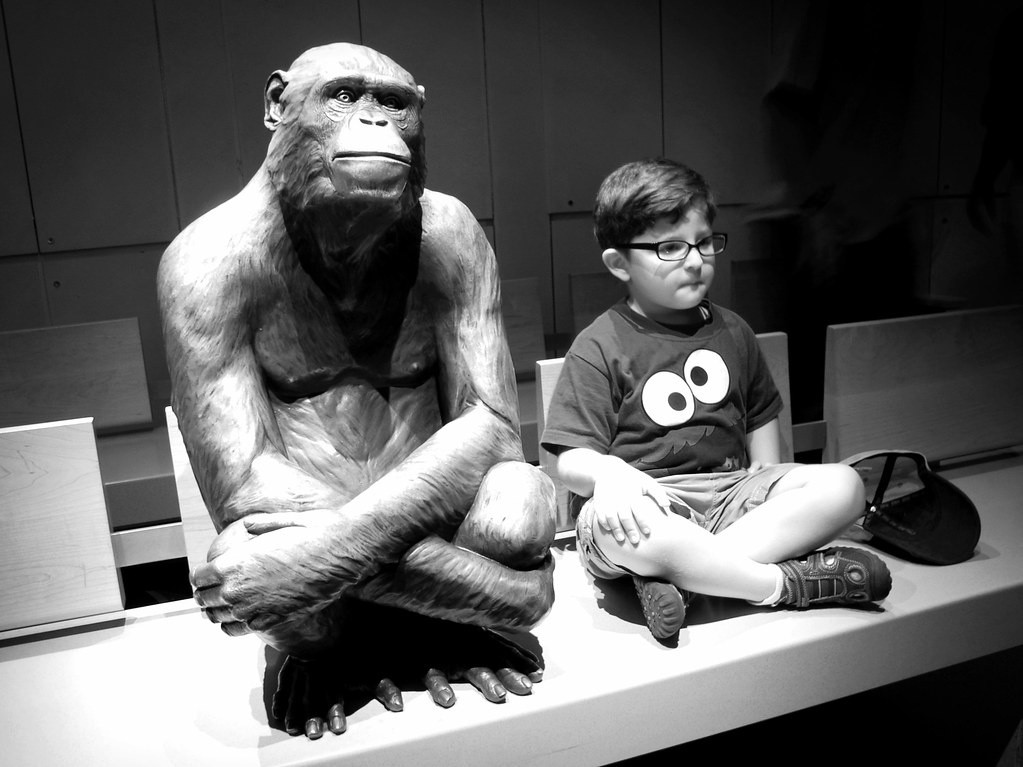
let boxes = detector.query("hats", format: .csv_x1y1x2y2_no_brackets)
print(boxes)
836,449,982,565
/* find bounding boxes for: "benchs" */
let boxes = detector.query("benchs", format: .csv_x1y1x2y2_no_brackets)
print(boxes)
1,304,1023,764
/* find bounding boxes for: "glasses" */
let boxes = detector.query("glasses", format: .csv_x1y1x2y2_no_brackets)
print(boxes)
617,231,729,262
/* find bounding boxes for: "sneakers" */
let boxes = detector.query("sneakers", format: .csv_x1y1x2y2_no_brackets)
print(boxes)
766,546,893,608
632,574,699,639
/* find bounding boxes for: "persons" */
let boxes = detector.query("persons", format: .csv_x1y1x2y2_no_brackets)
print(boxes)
537,156,892,639
